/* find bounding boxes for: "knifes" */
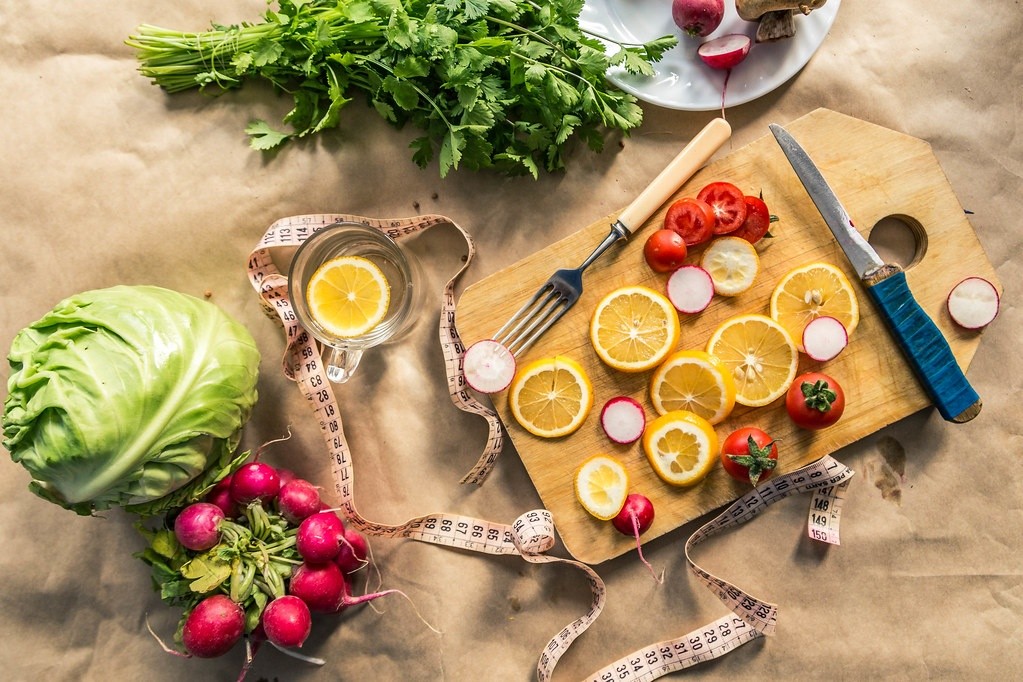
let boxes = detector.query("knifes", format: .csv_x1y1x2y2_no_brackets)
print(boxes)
767,122,981,425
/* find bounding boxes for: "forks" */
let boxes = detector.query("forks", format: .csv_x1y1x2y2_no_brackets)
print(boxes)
490,117,732,361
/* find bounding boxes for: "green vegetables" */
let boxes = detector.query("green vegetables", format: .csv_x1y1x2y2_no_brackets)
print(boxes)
122,0,677,181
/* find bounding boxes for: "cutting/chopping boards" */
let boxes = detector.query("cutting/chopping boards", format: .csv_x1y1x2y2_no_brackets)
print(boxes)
454,107,1006,563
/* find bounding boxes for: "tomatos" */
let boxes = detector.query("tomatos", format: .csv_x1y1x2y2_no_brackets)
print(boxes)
642,182,772,273
722,426,780,484
788,373,845,432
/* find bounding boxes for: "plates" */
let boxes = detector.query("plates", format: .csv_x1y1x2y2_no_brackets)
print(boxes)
575,0,842,110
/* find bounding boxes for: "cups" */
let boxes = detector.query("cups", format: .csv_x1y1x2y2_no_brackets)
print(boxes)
286,225,430,383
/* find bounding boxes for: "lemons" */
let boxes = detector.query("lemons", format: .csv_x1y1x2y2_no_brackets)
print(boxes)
507,353,593,440
575,236,861,522
307,256,390,338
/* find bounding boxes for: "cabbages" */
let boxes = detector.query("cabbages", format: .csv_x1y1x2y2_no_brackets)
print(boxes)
3,285,261,513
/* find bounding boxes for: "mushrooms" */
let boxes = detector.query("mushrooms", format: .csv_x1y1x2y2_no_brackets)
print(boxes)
735,0,829,43
